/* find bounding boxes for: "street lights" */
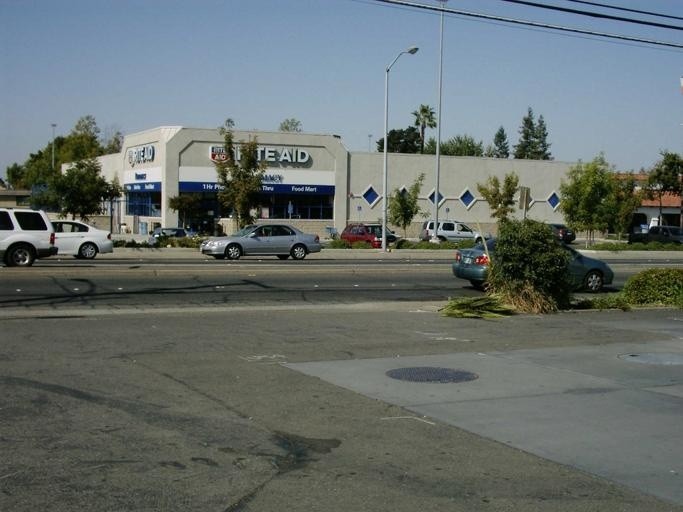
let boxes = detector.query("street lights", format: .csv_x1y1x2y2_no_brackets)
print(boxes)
50,122,58,169
381,45,421,249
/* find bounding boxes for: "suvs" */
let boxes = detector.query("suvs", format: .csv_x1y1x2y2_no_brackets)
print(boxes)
0,208,59,268
417,219,491,244
341,222,400,249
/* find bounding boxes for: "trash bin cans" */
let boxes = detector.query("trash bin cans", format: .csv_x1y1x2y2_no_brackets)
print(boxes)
213,223,220,237
141,222,148,235
153,222,161,234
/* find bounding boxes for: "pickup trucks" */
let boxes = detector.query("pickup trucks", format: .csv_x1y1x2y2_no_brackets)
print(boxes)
626,224,682,246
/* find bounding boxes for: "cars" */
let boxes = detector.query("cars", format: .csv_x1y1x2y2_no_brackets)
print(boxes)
45,218,115,259
548,223,577,244
199,223,322,261
147,228,188,243
189,225,226,238
452,237,614,292
